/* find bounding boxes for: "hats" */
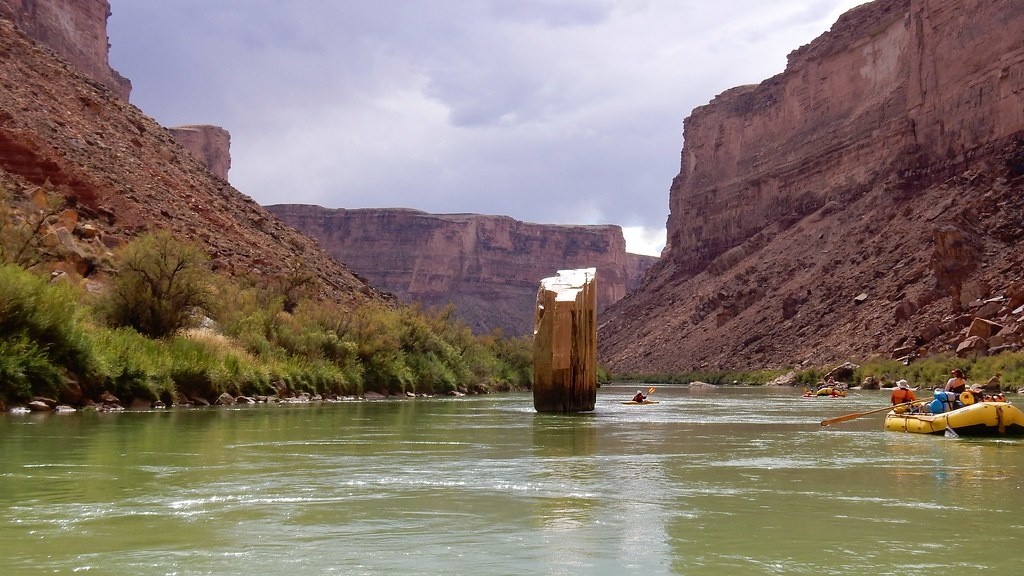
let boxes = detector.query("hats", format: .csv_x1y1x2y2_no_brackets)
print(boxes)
896,379,910,388
637,390,642,395
951,369,962,376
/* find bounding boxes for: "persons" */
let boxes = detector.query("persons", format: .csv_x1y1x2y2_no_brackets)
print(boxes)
890,369,970,412
810,376,847,395
633,390,647,402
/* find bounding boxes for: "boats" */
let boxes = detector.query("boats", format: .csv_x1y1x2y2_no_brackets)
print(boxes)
620,400,659,405
884,383,1024,440
816,382,846,397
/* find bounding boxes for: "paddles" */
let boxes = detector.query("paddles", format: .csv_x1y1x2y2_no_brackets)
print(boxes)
647,387,656,395
820,397,934,427
